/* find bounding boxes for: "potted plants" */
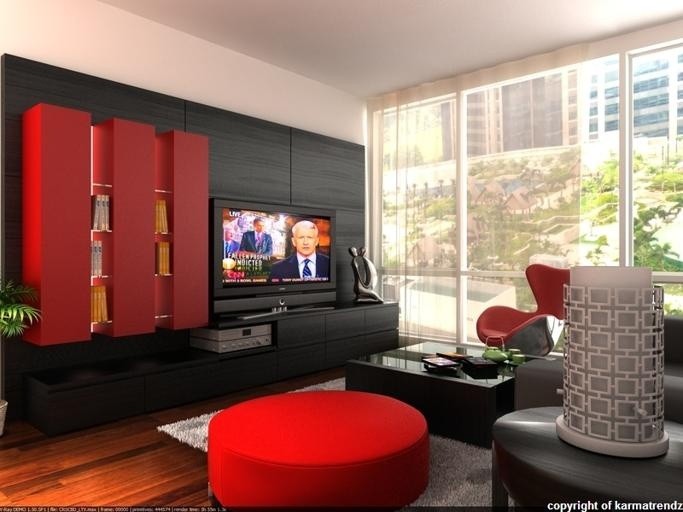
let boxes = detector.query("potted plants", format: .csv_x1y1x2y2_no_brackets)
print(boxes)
0,271,43,437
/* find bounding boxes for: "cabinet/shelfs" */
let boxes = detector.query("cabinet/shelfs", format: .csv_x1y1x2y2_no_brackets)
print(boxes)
91,184,112,324
16,303,400,440
155,189,173,320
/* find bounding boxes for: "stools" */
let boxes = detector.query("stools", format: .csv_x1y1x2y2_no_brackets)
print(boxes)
206,390,429,510
493,404,683,512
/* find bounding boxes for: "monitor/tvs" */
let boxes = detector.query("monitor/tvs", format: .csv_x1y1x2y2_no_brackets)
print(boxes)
213,199,338,320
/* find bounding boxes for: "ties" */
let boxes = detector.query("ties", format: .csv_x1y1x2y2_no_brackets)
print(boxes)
303,260,312,278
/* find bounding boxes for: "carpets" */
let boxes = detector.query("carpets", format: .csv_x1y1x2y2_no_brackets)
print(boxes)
156,375,517,510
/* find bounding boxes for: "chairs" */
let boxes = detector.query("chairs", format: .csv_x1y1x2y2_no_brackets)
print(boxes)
476,263,571,357
515,314,683,424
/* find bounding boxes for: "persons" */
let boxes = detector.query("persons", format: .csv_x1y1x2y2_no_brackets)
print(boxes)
225,228,240,258
268,220,329,278
238,218,273,259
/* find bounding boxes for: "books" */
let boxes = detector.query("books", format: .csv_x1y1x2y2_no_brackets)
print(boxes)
421,351,499,372
91,285,108,323
91,194,110,230
154,199,169,232
156,241,170,274
91,240,103,275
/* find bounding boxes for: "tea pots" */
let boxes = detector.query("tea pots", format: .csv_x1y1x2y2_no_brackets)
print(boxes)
482,336,507,363
